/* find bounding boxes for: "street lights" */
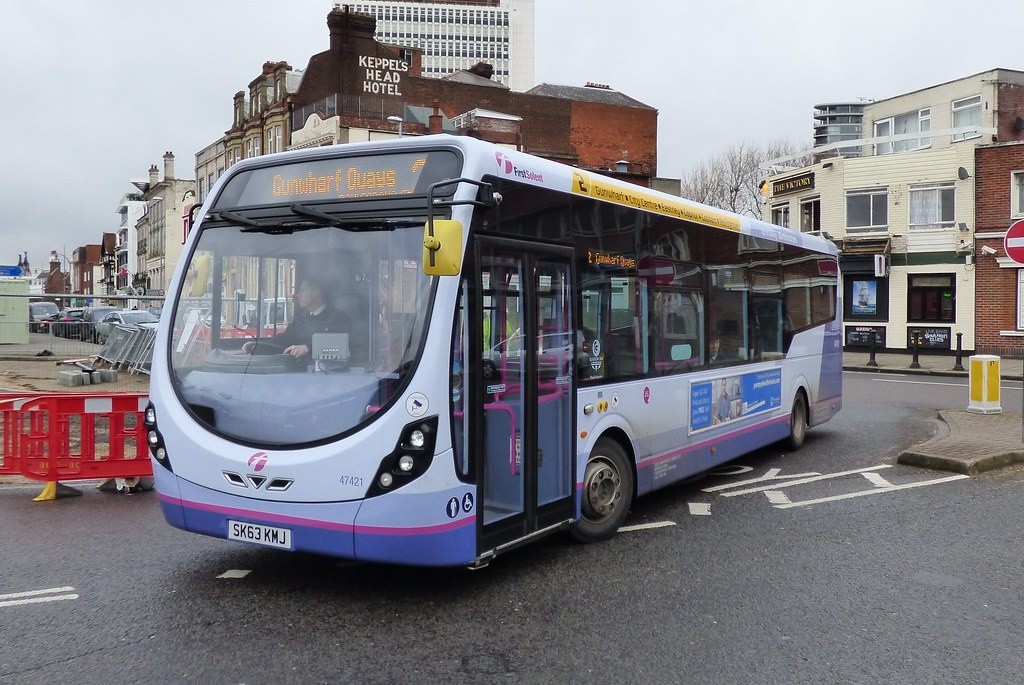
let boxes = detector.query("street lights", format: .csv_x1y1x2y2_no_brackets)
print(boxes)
387,116,402,139
49,254,71,307
34,267,45,298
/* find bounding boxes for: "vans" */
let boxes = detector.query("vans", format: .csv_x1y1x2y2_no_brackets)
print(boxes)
29,302,59,333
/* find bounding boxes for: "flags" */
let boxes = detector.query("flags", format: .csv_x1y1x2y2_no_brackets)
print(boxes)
120,267,126,277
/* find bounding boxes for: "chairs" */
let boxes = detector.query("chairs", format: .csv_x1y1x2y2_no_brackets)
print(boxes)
329,292,368,362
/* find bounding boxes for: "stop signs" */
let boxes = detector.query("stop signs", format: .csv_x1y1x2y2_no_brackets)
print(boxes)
636,255,676,288
1004,219,1024,265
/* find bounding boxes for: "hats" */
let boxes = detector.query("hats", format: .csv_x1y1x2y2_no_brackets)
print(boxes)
696,329,721,339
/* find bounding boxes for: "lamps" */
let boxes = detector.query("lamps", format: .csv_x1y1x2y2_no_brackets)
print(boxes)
823,163,833,168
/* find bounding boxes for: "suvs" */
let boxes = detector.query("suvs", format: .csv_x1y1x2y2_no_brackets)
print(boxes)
183,307,225,329
53,307,84,338
79,306,118,343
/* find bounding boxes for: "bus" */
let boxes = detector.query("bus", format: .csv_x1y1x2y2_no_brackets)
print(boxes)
142,131,844,571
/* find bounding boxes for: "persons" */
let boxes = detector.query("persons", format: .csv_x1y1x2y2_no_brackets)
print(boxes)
709,334,727,362
242,277,349,359
713,378,733,425
131,306,138,310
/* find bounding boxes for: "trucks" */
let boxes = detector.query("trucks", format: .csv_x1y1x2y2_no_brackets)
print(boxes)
244,297,293,330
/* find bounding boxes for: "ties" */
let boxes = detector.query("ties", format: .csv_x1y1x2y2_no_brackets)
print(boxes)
311,314,314,318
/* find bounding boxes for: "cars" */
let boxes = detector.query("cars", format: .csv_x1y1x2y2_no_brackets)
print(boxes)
476,324,600,379
145,307,163,319
94,310,160,345
